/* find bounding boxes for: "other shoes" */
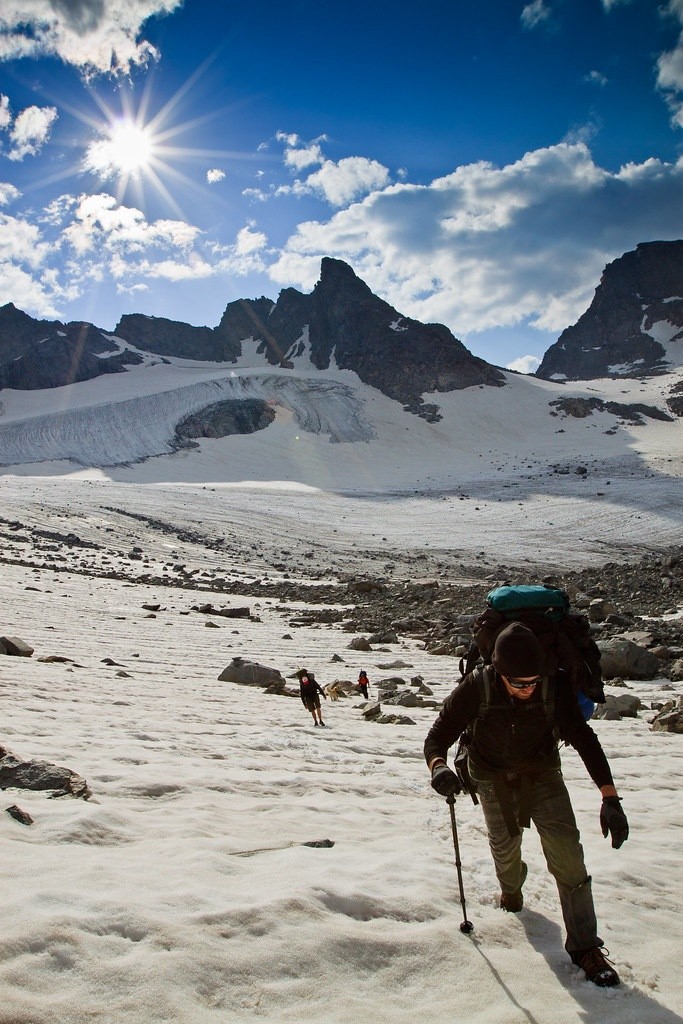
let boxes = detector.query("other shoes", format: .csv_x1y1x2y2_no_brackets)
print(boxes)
500,863,527,913
568,946,620,988
315,721,318,726
320,721,325,726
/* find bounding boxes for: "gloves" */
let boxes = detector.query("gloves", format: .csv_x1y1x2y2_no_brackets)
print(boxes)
431,765,461,796
600,796,629,849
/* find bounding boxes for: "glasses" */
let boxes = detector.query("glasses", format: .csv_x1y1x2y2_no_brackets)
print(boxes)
505,675,543,689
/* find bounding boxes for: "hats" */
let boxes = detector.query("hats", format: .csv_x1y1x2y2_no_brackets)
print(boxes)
491,622,544,677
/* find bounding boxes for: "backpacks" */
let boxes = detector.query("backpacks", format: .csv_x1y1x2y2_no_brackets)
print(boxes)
301,674,314,690
456,585,604,837
358,671,366,681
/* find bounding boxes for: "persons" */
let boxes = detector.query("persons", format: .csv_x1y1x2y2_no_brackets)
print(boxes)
359,671,371,700
298,673,327,726
421,621,631,989
